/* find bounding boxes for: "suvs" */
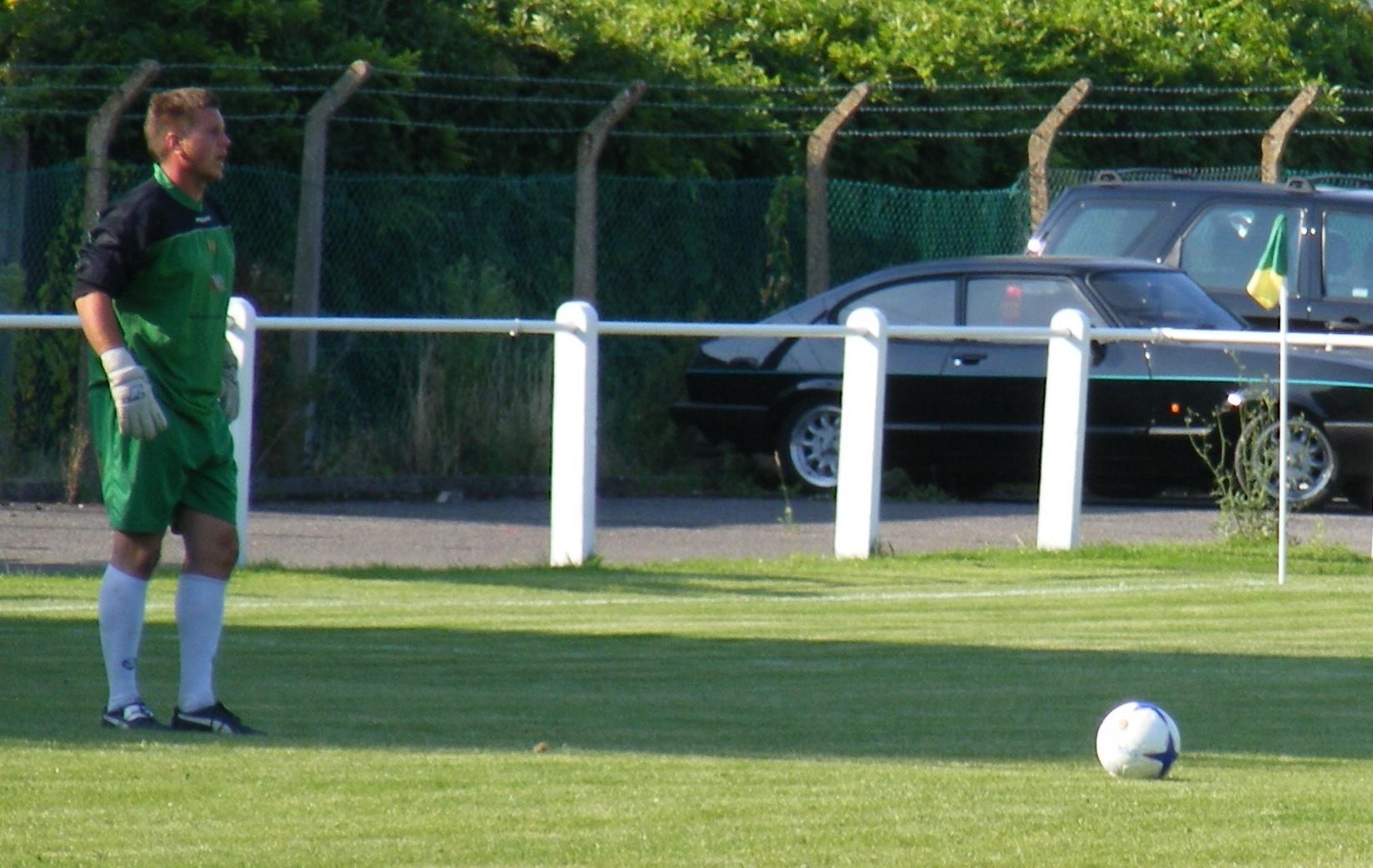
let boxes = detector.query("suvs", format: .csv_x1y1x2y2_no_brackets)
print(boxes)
1004,171,1372,362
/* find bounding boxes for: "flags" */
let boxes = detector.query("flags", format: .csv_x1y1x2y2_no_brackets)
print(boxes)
1247,212,1288,309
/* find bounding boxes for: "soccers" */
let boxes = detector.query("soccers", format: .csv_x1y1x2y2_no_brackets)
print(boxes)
1095,702,1180,779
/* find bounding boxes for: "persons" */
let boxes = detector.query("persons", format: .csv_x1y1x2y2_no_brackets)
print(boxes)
72,88,264,737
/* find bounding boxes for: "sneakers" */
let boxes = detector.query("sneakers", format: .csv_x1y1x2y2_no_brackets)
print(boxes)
103,699,171,733
171,698,269,737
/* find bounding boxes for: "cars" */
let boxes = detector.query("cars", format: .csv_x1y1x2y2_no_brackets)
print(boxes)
668,253,1370,511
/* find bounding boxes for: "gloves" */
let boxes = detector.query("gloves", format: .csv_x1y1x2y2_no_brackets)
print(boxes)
100,347,167,440
216,341,241,424
